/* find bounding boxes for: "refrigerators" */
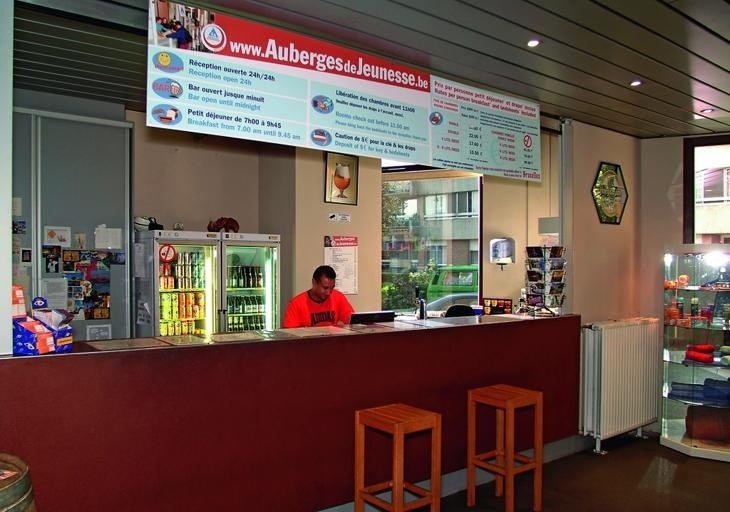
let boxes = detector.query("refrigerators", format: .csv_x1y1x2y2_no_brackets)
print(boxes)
133,229,282,338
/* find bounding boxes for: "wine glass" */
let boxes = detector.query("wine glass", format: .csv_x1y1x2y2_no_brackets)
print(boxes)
333,162,353,199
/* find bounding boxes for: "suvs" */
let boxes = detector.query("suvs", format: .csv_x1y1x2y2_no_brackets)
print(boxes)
427,262,478,311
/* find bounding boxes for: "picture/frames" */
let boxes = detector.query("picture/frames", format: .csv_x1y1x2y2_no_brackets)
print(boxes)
323,150,360,207
589,160,628,227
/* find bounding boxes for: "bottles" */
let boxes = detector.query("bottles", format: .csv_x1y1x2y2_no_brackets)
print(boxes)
225,264,266,333
676,297,699,318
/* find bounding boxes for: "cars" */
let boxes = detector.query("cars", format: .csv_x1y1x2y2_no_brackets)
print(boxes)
424,294,477,312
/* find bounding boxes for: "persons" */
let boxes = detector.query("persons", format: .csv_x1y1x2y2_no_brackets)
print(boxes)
283,263,357,328
155,15,193,51
47,258,58,272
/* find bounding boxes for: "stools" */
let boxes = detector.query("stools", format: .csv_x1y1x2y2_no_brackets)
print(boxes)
351,401,444,512
464,381,546,512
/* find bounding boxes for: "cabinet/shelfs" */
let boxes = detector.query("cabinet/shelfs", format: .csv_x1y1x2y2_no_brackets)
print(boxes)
657,244,730,466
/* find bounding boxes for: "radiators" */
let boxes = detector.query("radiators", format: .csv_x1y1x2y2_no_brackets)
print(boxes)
576,315,663,457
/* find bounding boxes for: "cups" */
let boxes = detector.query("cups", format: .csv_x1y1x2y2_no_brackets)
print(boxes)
170,86,183,96
